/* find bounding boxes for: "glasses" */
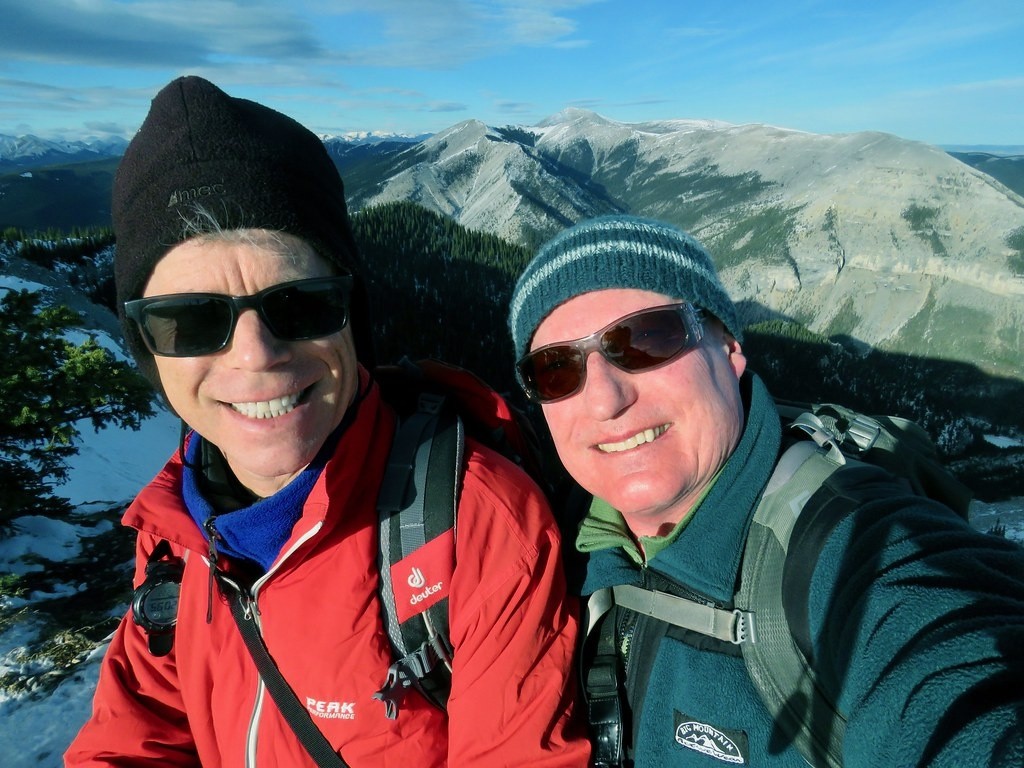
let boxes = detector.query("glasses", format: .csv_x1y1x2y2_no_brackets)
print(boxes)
124,259,355,358
515,301,715,404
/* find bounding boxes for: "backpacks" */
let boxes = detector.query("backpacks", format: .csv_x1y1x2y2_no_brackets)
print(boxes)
575,395,975,768
370,354,569,714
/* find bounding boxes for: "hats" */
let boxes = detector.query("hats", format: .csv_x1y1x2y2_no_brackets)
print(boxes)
110,74,360,419
506,214,746,403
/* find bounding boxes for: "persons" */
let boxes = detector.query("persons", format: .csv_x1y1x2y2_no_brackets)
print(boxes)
506,212,1024,768
63,74,591,768
